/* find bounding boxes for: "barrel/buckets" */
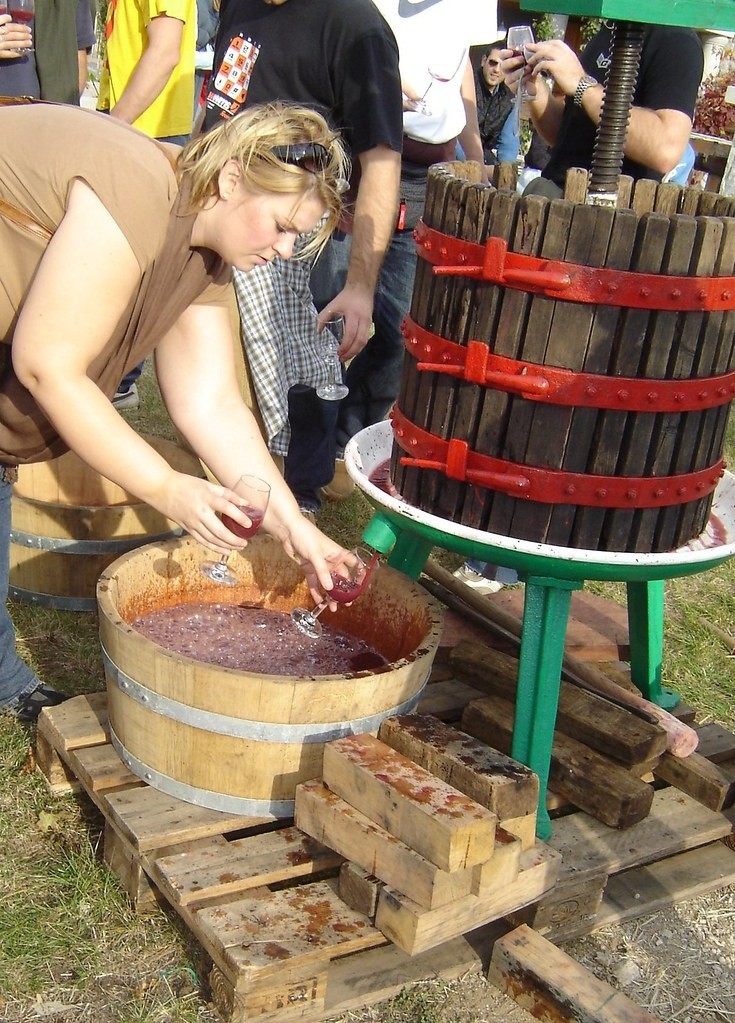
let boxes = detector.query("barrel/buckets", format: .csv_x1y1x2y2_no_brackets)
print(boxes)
95,525,443,819
8,434,221,611
388,159,735,554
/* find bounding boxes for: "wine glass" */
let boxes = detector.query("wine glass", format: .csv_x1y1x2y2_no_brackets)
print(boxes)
507,25,538,103
205,476,276,584
292,549,384,637
316,312,350,401
7,0,36,53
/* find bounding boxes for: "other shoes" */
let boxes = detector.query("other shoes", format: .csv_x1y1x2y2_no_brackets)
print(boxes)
299,509,316,526
321,455,356,500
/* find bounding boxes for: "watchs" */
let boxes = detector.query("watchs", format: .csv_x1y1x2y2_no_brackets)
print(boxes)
573,75,598,107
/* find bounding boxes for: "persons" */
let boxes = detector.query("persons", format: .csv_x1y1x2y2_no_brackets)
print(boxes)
0,0,98,106
96,0,197,410
199,0,403,513
455,44,518,165
516,118,553,197
319,0,497,500
0,95,374,722
664,143,696,186
193,0,221,118
450,17,704,597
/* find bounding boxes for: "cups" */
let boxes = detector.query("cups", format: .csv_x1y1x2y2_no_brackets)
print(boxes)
0,0,8,29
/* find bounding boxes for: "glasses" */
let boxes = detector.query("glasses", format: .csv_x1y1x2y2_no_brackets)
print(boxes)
486,58,498,66
254,142,332,174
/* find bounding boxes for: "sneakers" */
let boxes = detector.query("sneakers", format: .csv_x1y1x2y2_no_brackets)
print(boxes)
112,383,140,411
452,564,505,596
0,683,70,720
